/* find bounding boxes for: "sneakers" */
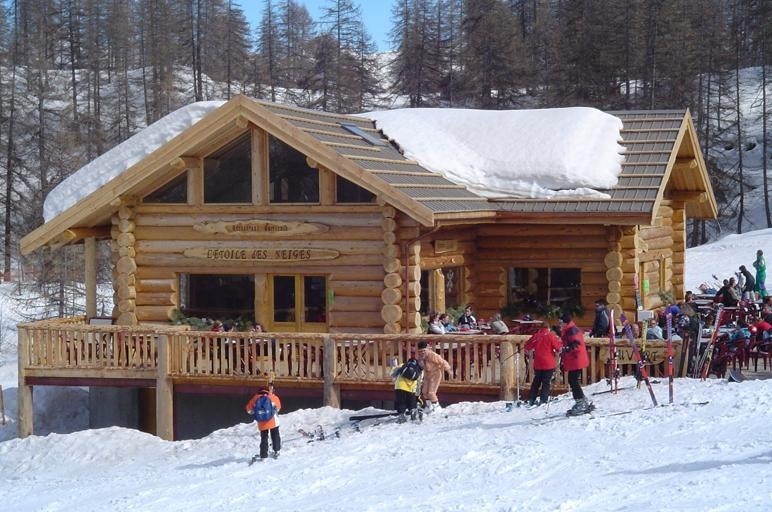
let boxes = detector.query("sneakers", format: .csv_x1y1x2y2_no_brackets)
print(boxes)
571,398,588,412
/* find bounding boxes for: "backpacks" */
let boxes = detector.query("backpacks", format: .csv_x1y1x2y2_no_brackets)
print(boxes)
399,362,422,381
253,397,276,421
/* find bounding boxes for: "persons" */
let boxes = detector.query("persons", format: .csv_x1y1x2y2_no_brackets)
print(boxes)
391,299,610,425
246,386,281,462
187,320,275,375
623,250,772,378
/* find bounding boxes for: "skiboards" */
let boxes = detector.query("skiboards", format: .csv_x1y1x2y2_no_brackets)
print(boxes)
248,411,423,465
608,308,724,406
498,380,708,421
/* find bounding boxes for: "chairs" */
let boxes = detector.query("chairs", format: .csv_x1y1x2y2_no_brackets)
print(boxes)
684,293,771,378
59,316,115,359
187,320,225,374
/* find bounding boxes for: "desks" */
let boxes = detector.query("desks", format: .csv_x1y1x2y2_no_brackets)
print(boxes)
225,340,374,377
125,333,158,366
427,320,544,381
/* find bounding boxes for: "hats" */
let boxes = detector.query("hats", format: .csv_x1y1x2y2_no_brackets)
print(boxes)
258,386,269,393
559,314,570,324
417,341,427,349
